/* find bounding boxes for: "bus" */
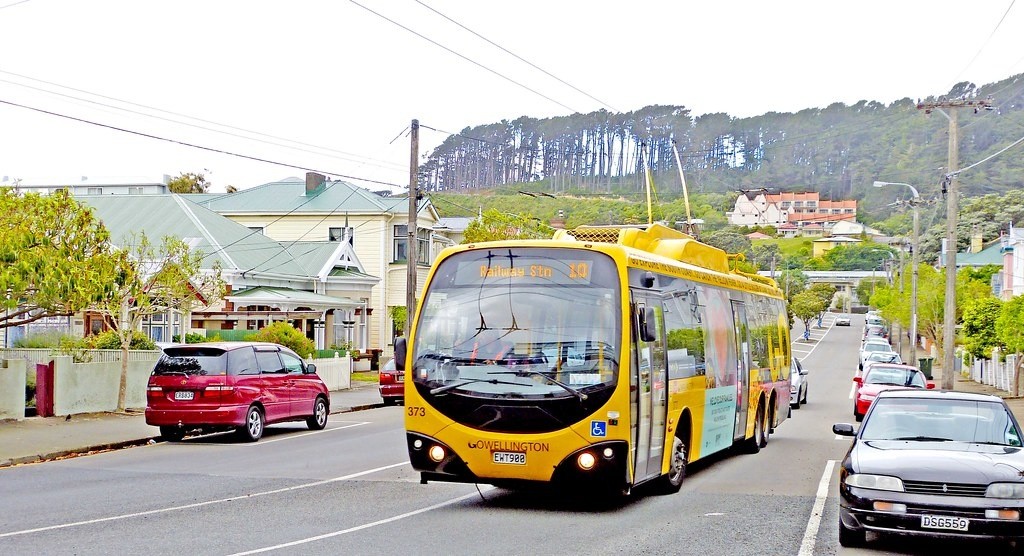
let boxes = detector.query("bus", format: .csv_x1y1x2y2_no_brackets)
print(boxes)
395,224,791,499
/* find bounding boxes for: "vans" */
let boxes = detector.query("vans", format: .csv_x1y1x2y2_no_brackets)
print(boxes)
146,339,330,442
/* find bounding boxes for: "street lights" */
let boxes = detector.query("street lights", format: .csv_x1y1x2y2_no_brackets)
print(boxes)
870,181,918,363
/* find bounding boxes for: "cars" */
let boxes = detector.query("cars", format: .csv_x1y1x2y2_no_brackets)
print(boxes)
790,357,809,408
859,311,906,372
833,388,1024,548
836,315,850,326
379,355,405,403
852,364,935,423
